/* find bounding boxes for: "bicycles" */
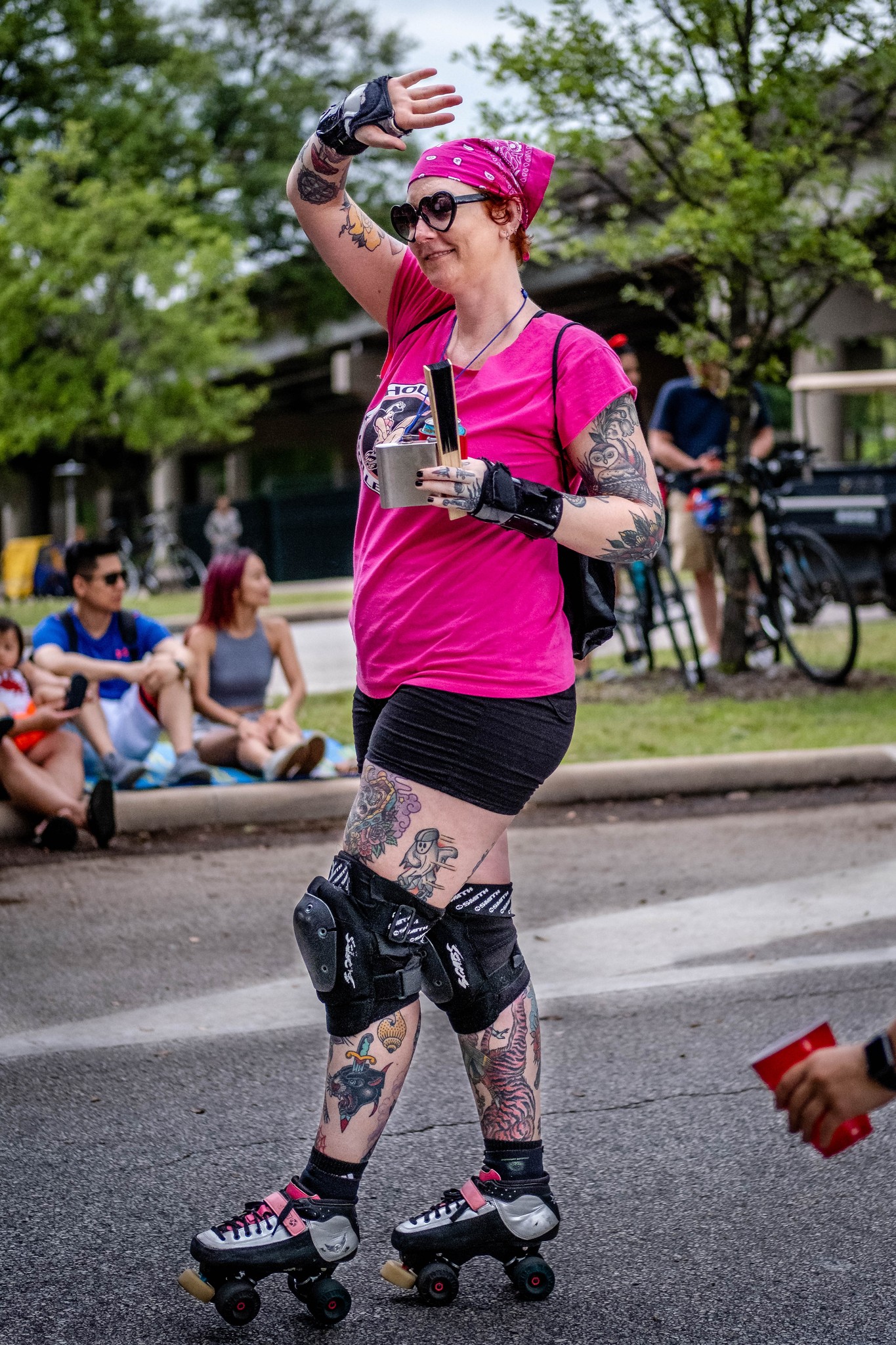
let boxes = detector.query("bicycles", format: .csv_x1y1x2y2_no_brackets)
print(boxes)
709,444,863,686
610,465,708,692
140,500,210,599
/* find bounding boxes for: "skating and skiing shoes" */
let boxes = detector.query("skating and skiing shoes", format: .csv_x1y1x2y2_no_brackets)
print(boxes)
391,1147,561,1307
191,1161,359,1326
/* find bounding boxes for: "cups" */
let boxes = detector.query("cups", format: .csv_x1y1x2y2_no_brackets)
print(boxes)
748,1019,873,1159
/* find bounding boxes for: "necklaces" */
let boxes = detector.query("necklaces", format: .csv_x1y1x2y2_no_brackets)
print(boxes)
398,288,528,442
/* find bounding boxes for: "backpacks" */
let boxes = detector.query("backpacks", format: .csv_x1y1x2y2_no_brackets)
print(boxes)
387,297,618,663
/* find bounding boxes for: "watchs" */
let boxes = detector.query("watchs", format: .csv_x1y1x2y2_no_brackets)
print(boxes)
861,1028,896,1093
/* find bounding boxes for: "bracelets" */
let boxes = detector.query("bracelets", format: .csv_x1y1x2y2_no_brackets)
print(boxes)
172,658,186,682
233,716,247,728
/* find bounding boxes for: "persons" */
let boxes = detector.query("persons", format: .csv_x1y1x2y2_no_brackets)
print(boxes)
648,328,777,669
183,548,326,783
205,492,243,553
0,615,89,753
0,659,116,852
190,67,666,1271
772,1023,896,1146
31,534,212,791
573,333,664,681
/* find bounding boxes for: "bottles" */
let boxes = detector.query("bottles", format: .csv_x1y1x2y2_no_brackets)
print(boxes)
374,435,442,508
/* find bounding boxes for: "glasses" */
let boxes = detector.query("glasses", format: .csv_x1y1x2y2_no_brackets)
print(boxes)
390,190,491,244
78,569,128,585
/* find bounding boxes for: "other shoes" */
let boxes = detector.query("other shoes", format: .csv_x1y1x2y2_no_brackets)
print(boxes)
580,659,652,684
29,774,118,852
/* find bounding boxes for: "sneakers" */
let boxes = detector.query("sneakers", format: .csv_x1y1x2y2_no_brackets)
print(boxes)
262,734,324,782
744,631,777,672
168,750,213,787
106,752,147,788
683,647,721,672
